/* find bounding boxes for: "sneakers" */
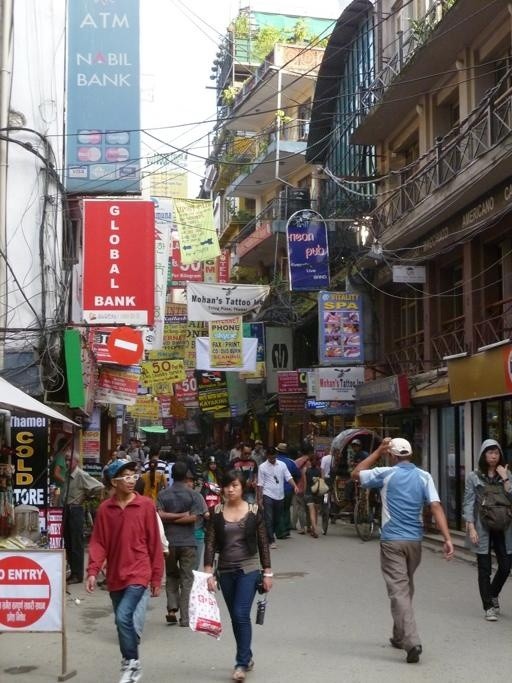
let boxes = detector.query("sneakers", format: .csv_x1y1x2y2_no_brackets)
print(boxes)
291,524,319,539
96,578,108,590
269,542,277,550
490,595,501,613
406,643,422,663
388,637,403,649
66,576,85,585
118,658,144,683
484,607,497,620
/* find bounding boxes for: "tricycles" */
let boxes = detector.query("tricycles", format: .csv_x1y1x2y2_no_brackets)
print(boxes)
317,425,382,542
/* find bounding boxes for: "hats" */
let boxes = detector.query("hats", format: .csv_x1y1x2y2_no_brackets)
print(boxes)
275,442,289,452
385,436,413,457
105,458,138,477
205,455,218,465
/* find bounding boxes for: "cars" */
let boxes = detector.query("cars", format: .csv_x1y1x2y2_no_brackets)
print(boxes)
158,445,172,455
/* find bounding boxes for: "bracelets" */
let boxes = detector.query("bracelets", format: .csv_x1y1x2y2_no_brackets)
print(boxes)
501,477,510,483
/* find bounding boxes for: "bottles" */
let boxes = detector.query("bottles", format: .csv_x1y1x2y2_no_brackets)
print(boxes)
256,590,266,625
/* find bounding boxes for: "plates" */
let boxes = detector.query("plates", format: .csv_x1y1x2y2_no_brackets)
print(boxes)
325,336,360,359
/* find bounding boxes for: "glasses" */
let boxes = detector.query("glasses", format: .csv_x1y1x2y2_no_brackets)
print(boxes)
112,472,140,482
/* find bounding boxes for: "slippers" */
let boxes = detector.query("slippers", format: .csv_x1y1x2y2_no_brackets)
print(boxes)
165,613,178,624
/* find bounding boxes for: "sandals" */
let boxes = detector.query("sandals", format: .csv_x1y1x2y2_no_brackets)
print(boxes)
246,654,254,670
232,665,246,682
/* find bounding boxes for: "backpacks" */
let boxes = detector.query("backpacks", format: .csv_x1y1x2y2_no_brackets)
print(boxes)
474,469,512,531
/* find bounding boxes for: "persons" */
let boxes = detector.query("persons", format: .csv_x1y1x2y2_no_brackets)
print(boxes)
462,438,512,621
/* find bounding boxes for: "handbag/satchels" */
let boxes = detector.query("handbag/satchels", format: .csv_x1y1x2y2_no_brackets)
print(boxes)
310,476,328,497
257,569,267,595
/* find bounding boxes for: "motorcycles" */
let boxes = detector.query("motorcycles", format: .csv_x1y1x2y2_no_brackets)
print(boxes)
203,492,220,507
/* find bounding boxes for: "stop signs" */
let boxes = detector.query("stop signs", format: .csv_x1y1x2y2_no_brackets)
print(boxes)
0,556,52,628
107,326,143,366
